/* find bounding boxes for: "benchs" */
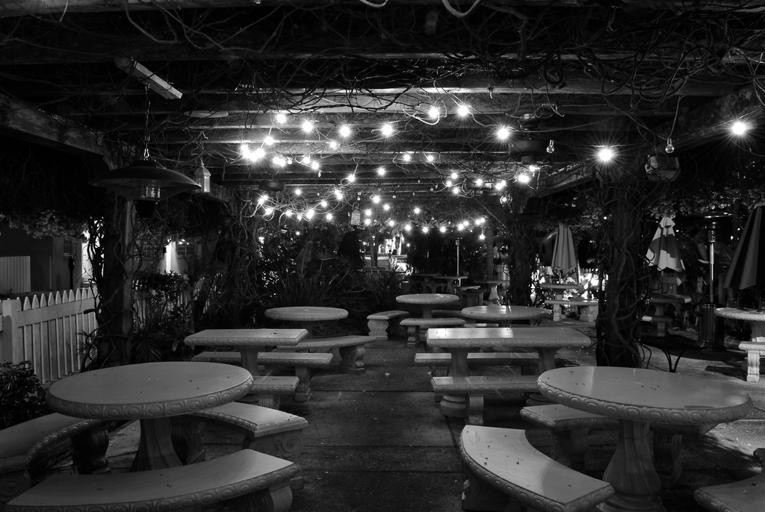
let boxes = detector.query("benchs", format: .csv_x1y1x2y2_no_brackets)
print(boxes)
0,280,765,512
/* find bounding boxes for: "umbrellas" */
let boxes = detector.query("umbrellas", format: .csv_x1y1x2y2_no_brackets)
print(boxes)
725,203,765,308
644,213,686,294
552,221,577,286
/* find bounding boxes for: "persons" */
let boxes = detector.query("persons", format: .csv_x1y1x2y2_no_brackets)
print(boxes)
487,284,506,306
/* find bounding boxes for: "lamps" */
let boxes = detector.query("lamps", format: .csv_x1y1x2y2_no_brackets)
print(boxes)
192,155,212,197
94,53,204,204
